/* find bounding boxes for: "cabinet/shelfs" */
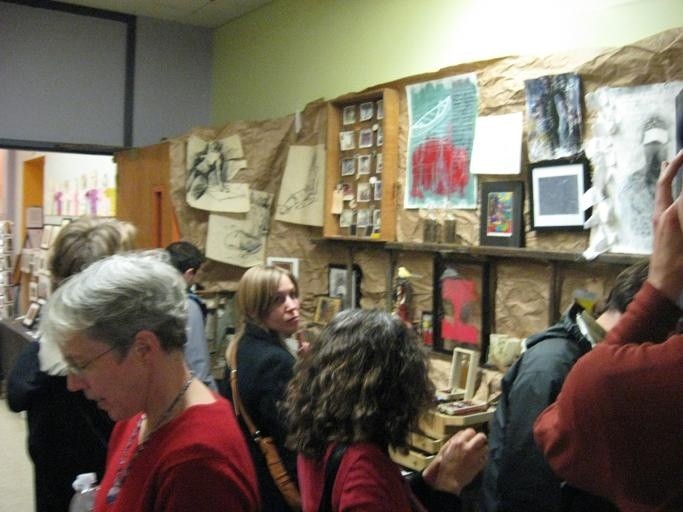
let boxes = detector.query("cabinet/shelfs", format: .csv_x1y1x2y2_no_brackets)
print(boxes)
387,385,495,477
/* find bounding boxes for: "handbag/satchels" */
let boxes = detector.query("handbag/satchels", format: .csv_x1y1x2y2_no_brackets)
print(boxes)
256,430,300,510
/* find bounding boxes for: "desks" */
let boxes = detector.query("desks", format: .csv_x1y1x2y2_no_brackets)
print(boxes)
0,319,36,380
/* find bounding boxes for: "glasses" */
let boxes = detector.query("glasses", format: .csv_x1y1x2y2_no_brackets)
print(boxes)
70,346,112,374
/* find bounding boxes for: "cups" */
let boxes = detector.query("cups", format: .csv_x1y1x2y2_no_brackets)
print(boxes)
486,332,526,367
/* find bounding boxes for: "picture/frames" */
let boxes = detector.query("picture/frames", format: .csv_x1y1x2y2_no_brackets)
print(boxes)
0,218,74,329
525,156,593,234
313,296,342,328
418,310,434,348
266,255,300,283
446,346,481,403
477,178,526,249
326,262,361,312
429,252,497,367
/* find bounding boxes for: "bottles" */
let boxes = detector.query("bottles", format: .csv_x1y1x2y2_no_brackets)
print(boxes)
65,472,100,511
421,203,457,244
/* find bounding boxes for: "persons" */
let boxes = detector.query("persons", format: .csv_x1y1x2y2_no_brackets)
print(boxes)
286,305,486,510
533,147,682,511
487,253,653,512
185,138,231,199
44,251,261,512
161,242,217,393
221,266,310,511
6,218,138,512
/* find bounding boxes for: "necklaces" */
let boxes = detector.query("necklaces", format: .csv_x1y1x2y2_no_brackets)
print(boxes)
102,365,196,505
